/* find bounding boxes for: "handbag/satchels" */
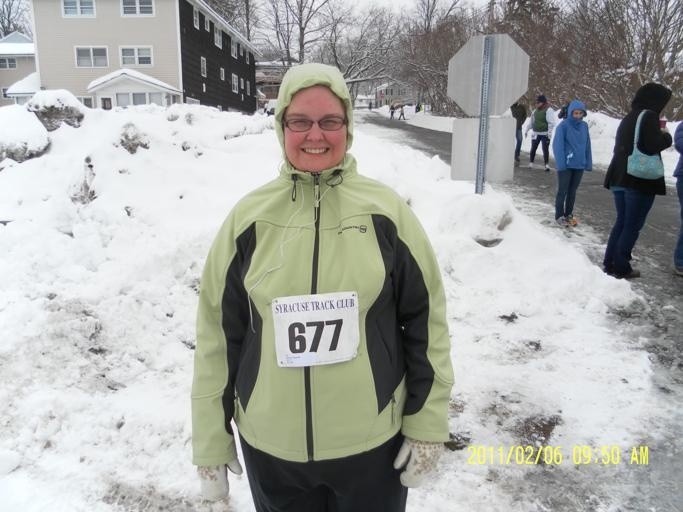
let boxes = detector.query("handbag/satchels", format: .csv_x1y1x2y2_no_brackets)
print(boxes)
626,147,664,180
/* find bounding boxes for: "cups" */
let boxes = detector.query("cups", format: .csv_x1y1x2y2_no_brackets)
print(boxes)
659,118,667,128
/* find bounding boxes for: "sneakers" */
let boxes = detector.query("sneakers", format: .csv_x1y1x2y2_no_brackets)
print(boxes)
671,267,683,276
528,162,533,169
624,269,641,278
556,215,569,226
566,214,577,226
544,164,550,171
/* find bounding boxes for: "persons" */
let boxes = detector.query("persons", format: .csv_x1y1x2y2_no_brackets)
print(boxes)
188,59,457,512
397,106,405,120
671,119,683,278
506,95,528,163
522,95,556,172
556,93,573,122
388,105,395,120
550,99,594,229
599,81,673,281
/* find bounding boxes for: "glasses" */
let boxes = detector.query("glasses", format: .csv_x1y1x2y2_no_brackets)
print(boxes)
282,116,347,133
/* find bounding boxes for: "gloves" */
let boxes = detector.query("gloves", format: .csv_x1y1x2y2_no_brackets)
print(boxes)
196,457,243,502
393,436,445,488
